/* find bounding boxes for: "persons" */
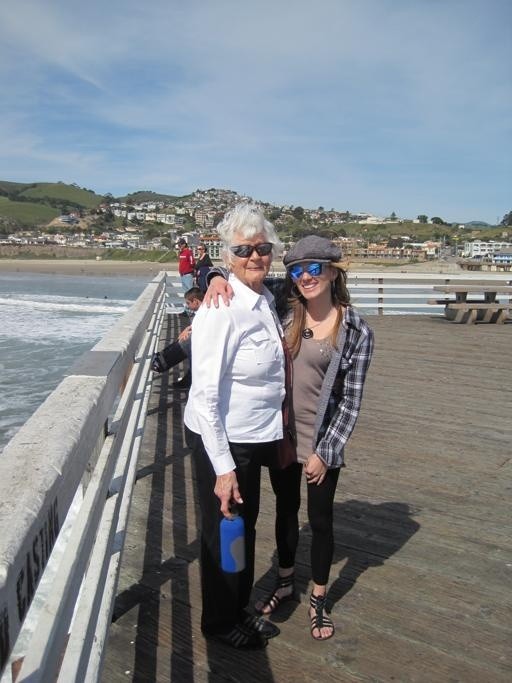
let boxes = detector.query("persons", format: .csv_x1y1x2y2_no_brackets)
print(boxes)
202,234,374,642
174,238,196,293
184,287,204,311
184,202,287,652
150,324,192,387
194,243,213,293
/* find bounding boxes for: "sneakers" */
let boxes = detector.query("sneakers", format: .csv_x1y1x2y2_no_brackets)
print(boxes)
206,610,279,650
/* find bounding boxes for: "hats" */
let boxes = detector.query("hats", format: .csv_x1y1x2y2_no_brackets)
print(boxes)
283,235,340,268
175,239,186,244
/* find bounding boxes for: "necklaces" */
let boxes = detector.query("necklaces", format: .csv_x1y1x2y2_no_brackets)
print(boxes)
302,307,333,339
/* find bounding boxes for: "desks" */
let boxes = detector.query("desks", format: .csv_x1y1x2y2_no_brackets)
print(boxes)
433,284,512,324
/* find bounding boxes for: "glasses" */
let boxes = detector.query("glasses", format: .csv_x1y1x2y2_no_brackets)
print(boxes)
289,263,322,279
230,243,272,257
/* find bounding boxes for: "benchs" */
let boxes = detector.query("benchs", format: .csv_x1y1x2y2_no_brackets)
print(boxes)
448,303,512,310
436,299,500,304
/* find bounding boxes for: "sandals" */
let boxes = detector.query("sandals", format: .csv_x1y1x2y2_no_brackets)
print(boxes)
308,590,334,640
254,575,297,615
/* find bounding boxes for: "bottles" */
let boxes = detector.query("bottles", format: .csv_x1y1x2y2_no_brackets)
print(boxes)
219,502,248,575
